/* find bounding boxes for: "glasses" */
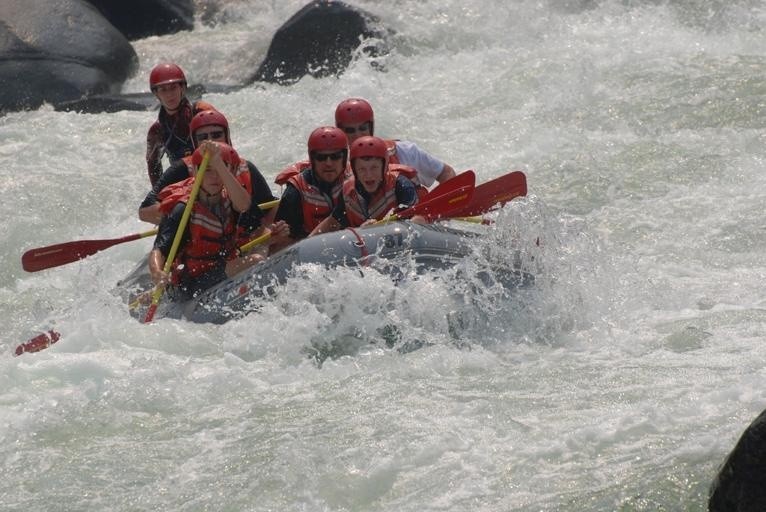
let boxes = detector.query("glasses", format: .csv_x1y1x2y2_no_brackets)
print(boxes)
195,130,224,141
343,120,371,133
312,150,345,161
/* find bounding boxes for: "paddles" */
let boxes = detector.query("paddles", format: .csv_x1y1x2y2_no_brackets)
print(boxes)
22,200,280,272
373,170,527,224
16,231,282,355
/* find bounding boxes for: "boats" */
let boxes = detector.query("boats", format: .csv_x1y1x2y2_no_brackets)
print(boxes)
115,222,535,325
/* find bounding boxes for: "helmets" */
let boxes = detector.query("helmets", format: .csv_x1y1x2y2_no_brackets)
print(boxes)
349,136,389,185
191,141,241,166
335,98,374,126
150,63,187,91
189,110,230,150
308,126,348,168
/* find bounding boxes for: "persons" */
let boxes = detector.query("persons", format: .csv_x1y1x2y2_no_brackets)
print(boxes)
139,109,289,301
268,97,457,255
146,62,218,188
